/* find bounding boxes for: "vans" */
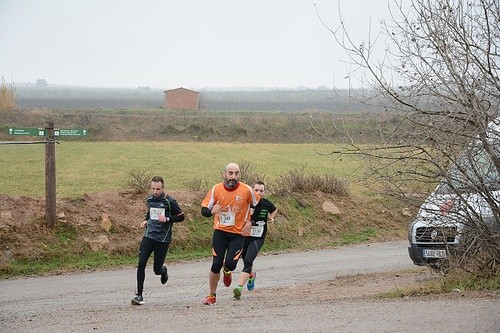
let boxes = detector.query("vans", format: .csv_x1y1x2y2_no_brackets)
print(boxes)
407,116,500,274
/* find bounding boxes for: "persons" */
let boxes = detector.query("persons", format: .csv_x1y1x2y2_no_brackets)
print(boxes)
131,176,185,304
233,180,277,300
200,162,263,304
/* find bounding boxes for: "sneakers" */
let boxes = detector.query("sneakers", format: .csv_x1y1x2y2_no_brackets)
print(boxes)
233,285,242,299
132,296,144,305
223,270,231,287
161,266,168,284
247,272,256,290
203,294,216,305
